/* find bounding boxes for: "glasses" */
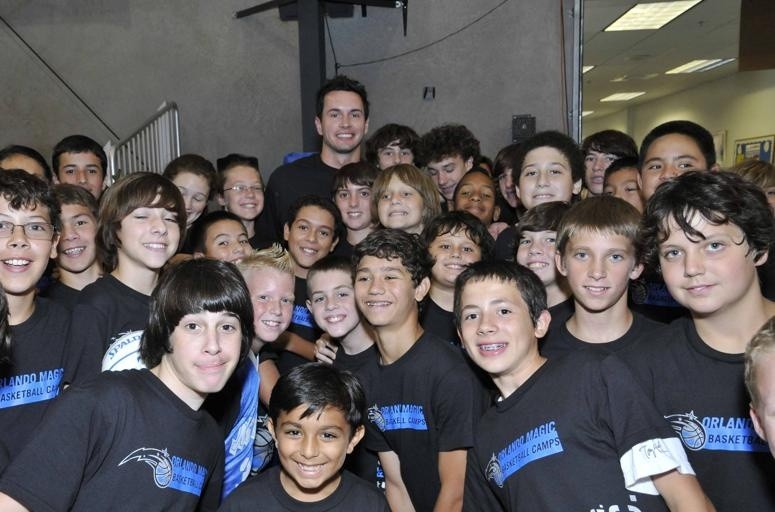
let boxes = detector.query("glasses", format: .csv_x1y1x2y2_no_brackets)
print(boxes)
0,221,57,241
223,183,267,196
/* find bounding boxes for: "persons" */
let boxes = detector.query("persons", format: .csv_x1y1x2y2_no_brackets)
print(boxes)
262,74,371,237
0,120,775,512
